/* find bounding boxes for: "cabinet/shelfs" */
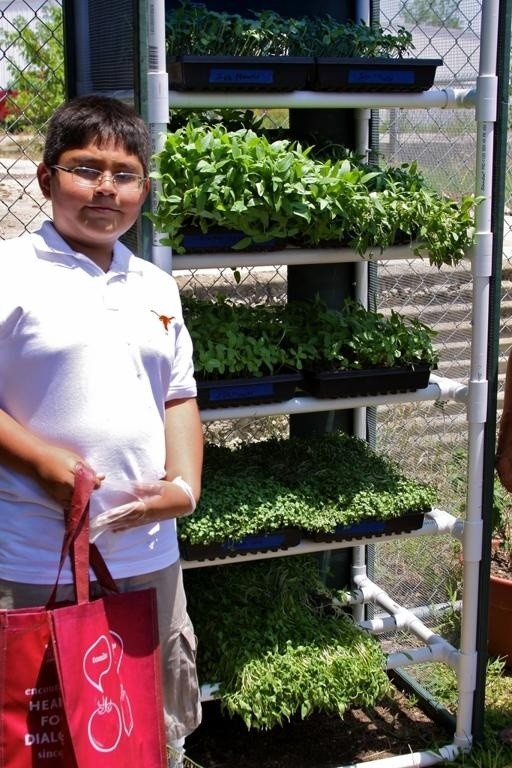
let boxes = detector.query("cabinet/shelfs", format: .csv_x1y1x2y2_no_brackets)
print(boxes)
76,3,499,768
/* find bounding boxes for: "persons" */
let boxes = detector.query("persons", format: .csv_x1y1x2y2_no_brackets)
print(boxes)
0,94,202,768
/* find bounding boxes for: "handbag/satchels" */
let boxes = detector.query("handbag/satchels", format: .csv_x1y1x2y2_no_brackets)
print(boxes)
0,586,167,767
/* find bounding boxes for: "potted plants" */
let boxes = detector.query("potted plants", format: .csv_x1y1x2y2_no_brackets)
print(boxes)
456,477,512,669
143,111,484,266
170,428,435,564
162,4,445,94
168,282,445,405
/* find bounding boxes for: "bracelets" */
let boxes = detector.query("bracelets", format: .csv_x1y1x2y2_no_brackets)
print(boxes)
169,477,197,521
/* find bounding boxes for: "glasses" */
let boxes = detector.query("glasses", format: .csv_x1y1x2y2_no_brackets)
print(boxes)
51,166,149,195
